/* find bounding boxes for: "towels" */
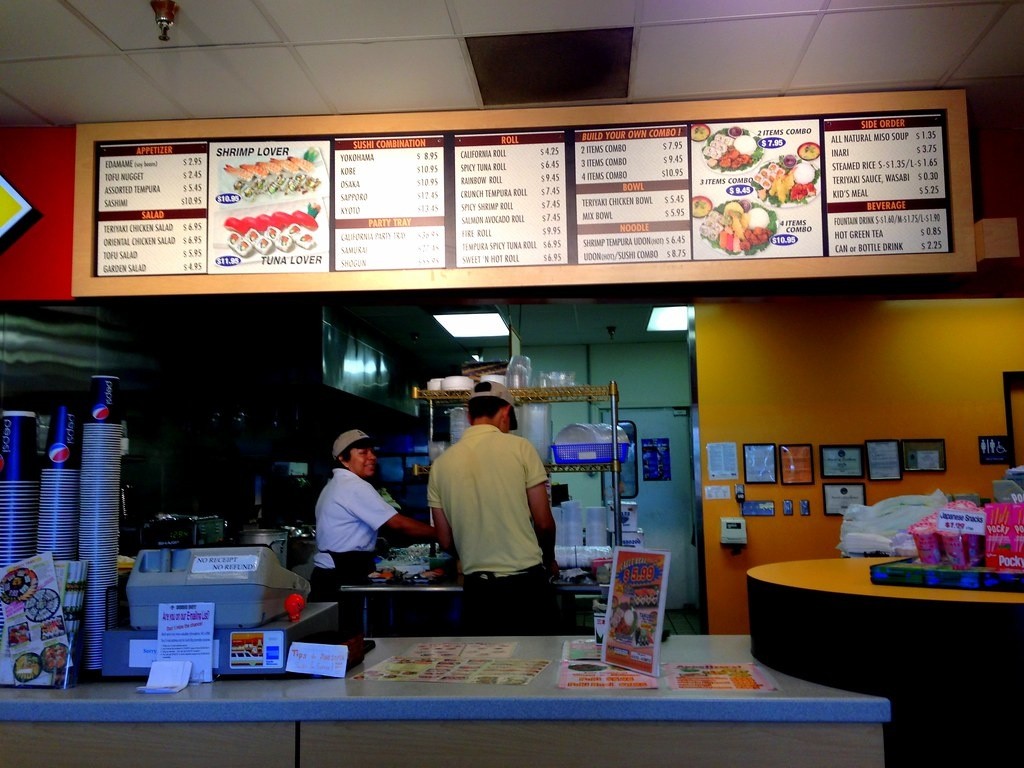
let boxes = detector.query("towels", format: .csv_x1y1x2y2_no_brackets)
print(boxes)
835,489,948,553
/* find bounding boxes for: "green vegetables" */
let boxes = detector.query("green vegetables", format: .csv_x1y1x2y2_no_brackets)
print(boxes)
751,156,821,208
702,128,764,172
699,201,778,255
568,664,606,671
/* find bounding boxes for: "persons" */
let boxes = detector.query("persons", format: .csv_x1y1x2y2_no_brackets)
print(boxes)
428,381,560,636
309,429,442,636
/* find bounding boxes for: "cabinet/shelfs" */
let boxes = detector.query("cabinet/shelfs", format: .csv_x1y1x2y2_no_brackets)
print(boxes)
411,380,622,549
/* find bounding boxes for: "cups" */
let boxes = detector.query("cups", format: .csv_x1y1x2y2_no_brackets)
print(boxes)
0,374,124,670
395,542,439,564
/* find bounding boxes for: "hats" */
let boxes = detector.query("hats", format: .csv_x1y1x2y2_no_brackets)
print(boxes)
332,429,369,462
468,381,518,430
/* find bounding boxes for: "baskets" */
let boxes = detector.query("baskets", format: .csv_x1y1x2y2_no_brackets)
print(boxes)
551,442,631,464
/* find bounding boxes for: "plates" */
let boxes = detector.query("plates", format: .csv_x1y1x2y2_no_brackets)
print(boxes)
411,577,429,583
426,374,508,390
698,127,821,259
553,422,630,458
372,578,389,582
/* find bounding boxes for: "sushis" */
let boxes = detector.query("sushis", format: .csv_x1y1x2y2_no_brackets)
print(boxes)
223,209,320,255
221,155,322,201
701,133,785,240
1,570,68,679
611,588,659,646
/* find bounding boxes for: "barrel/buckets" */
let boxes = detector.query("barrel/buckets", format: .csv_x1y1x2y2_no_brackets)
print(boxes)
240,529,288,568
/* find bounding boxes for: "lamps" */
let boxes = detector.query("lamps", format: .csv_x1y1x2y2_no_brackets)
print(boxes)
430,303,510,336
645,302,692,332
151,0,179,40
